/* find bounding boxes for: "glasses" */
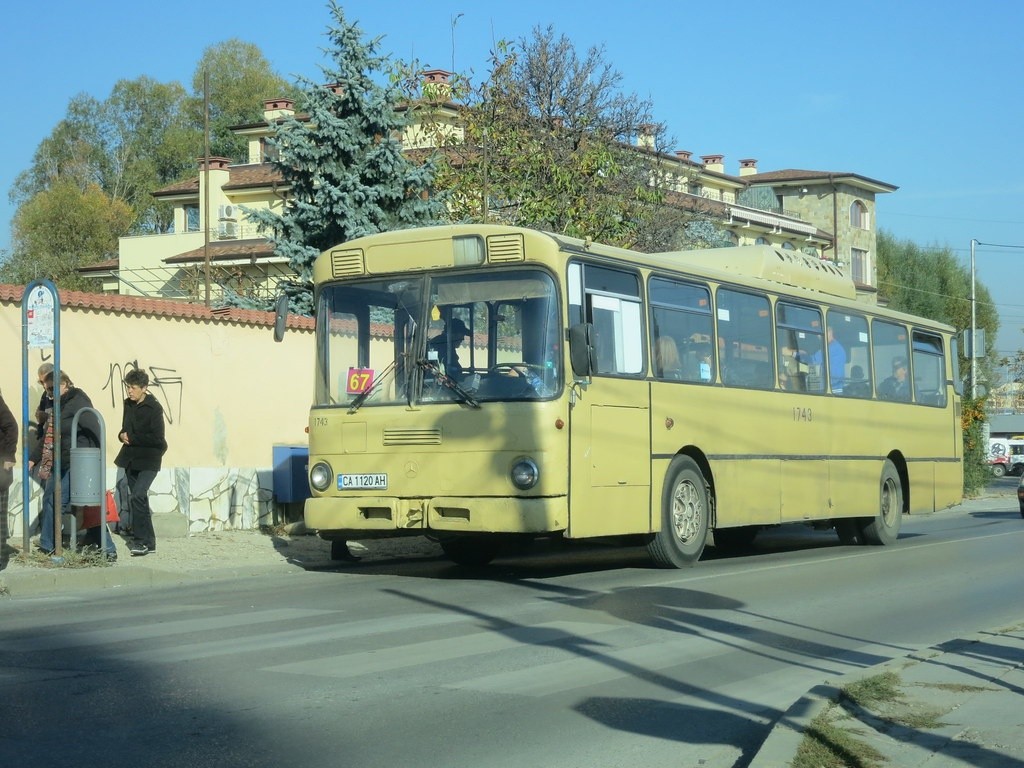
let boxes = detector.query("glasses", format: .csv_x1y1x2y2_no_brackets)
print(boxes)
48,386,54,392
38,381,44,386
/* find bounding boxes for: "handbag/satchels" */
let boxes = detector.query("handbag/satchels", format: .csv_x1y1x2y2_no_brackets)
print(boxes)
78,490,121,530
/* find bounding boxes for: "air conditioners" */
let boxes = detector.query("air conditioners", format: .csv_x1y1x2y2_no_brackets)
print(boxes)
219,222,239,239
218,205,238,220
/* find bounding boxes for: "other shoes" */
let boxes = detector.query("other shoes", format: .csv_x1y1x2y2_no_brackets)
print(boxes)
37,547,53,557
77,536,96,546
33,539,43,547
107,551,118,562
130,542,156,555
331,551,363,561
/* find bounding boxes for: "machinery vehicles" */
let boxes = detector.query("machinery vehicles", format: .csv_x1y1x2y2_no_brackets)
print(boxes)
986,441,1024,478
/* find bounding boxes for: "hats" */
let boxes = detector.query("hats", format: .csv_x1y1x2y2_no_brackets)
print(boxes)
445,318,471,335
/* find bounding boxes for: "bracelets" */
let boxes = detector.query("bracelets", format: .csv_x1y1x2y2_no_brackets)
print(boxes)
792,351,796,359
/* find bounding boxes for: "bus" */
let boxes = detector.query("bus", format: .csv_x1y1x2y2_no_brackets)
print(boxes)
271,221,968,569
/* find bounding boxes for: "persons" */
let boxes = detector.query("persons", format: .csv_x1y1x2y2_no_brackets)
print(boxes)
114,368,167,555
782,316,847,392
695,342,725,382
0,392,18,571
432,318,471,378
655,336,682,378
878,355,920,402
29,362,118,562
